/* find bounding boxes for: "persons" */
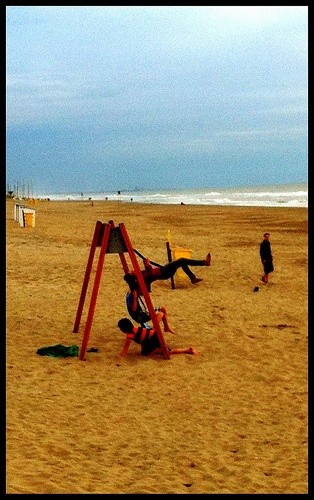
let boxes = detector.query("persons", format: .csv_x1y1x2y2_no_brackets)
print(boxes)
126,282,175,335
118,318,195,357
260,233,274,283
124,253,211,289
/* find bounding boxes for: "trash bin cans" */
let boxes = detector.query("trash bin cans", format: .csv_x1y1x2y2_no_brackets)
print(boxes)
174,250,191,277
24,212,33,228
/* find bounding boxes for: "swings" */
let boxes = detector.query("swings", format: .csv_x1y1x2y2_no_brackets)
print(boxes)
134,248,177,273
